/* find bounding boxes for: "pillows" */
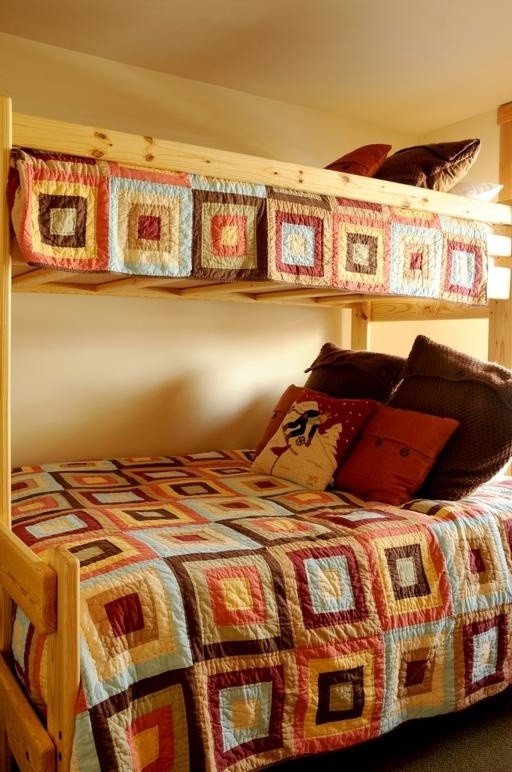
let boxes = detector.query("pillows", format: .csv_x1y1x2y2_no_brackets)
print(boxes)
332,403,459,506
321,137,504,203
252,391,375,494
389,337,512,503
253,334,409,460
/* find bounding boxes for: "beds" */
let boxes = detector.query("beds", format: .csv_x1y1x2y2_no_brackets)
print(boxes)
0,96,512,772
